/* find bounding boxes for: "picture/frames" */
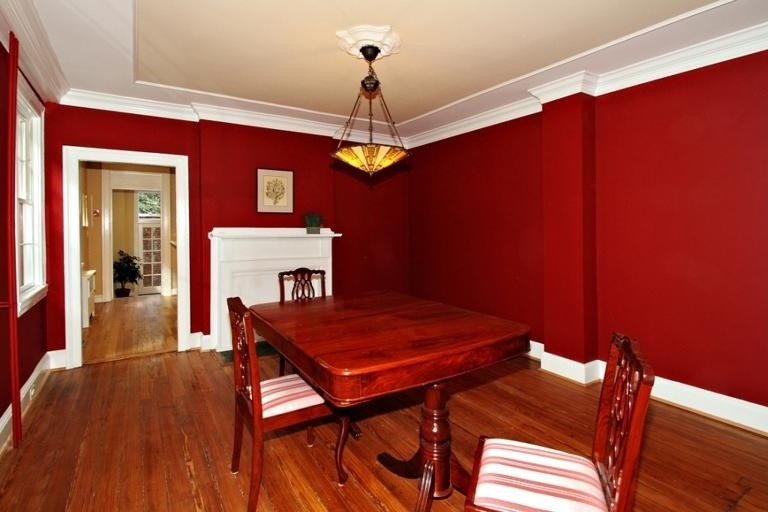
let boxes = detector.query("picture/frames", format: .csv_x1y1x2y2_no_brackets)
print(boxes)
255,167,294,214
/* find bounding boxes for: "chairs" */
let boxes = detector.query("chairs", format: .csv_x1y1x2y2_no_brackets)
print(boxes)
465,340,654,512
276,267,327,381
221,299,353,512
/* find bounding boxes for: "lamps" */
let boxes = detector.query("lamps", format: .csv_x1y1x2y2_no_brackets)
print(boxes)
332,23,413,178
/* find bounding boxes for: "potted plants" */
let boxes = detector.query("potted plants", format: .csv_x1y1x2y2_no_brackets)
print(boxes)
302,212,322,234
110,248,144,297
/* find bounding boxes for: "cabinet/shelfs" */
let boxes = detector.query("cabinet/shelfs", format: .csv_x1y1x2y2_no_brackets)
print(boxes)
81,269,96,328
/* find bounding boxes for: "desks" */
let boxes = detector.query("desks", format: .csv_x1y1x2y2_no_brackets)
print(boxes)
247,288,529,512
